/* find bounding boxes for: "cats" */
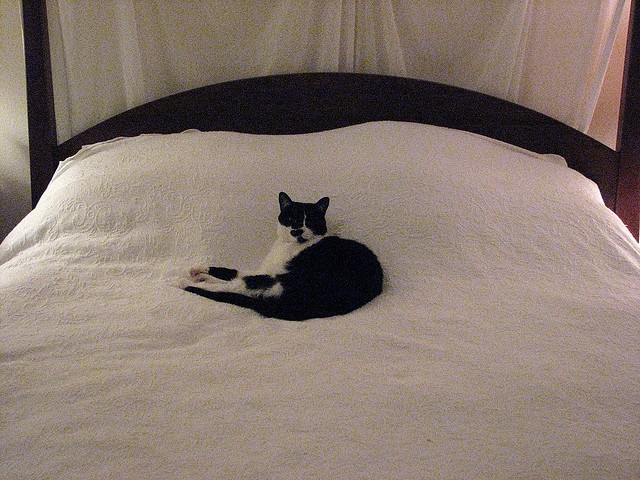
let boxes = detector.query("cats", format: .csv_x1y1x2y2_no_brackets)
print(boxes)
177,192,385,321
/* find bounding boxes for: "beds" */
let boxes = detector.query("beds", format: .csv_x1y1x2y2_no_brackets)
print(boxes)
0,0,640,480
